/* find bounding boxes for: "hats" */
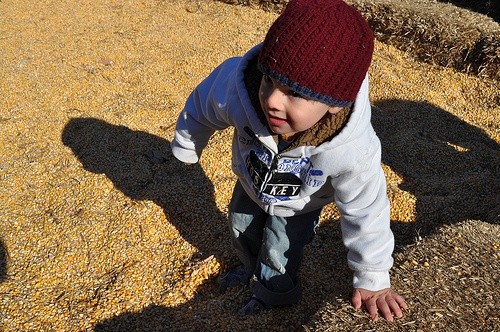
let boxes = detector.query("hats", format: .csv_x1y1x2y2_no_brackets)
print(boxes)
257,0,374,106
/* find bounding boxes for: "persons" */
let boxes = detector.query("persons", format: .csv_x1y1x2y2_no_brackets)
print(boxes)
171,0,411,323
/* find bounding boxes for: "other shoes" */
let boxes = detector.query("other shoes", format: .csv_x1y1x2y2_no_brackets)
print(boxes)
238,297,265,319
215,266,253,289
